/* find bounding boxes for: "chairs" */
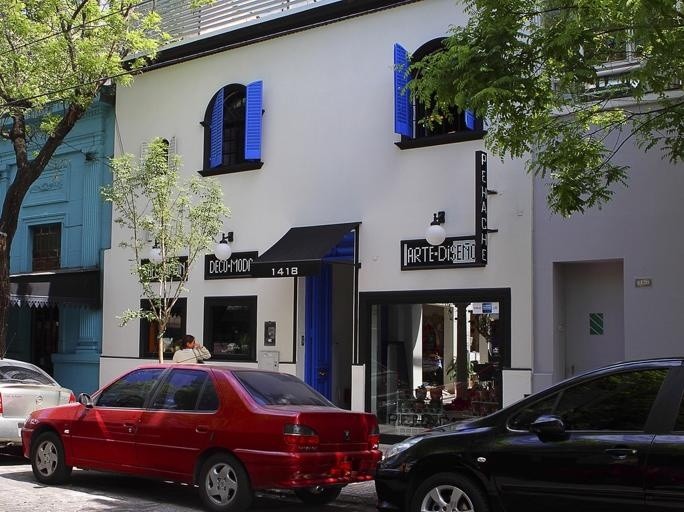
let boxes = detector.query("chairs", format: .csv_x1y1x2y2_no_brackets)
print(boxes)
174,386,198,410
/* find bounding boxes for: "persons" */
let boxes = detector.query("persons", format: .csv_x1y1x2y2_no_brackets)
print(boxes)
170,334,212,364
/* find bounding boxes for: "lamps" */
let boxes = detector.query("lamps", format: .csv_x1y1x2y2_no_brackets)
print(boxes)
148,237,165,265
214,232,233,260
426,211,446,246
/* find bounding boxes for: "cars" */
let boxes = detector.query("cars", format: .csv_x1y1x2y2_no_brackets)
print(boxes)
21,364,382,512
365,358,401,418
375,357,684,512
1,357,76,448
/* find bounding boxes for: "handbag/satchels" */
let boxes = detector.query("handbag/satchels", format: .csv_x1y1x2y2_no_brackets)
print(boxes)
192,349,204,363
